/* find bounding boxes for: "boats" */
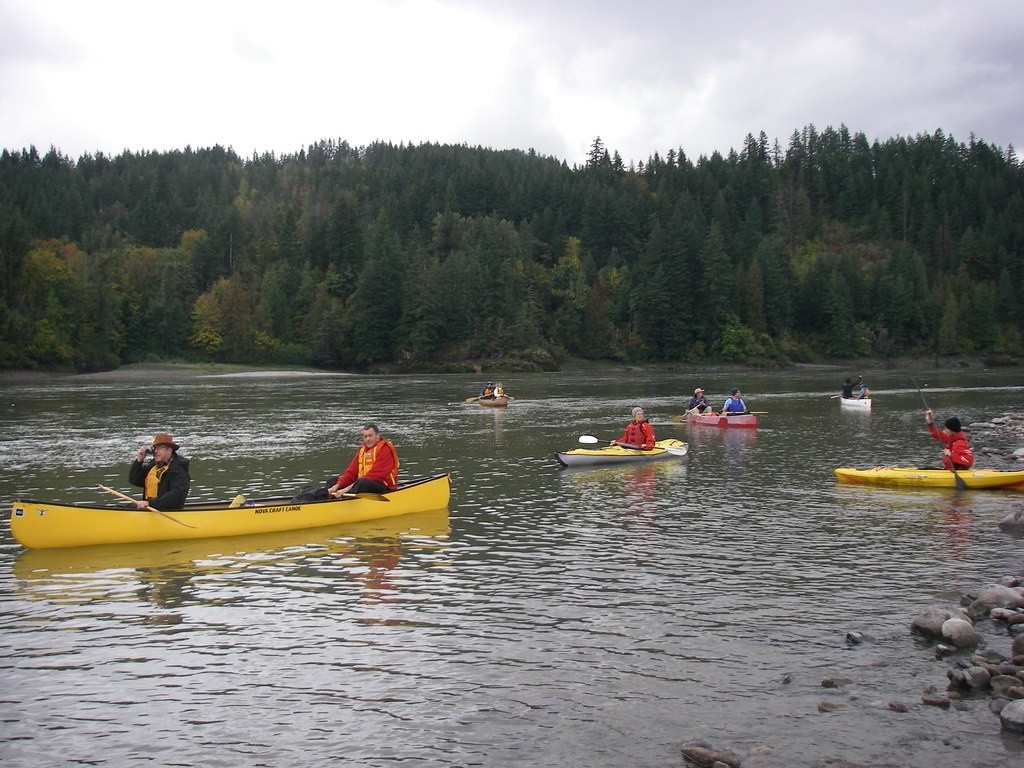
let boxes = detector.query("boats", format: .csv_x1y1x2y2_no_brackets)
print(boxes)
554,439,690,467
834,464,1024,489
14,508,449,581
841,396,871,408
686,410,758,430
479,396,508,407
11,473,452,550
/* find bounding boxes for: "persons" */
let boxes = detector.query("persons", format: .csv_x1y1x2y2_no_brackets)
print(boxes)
842,375,862,399
856,381,870,400
686,388,712,416
128,434,190,511
328,425,400,498
719,388,747,416
482,381,504,400
925,409,974,469
609,407,655,450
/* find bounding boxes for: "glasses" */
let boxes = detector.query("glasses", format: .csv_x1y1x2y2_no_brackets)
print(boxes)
151,446,170,451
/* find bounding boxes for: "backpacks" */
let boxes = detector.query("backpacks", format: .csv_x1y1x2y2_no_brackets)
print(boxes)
865,389,870,396
290,484,329,503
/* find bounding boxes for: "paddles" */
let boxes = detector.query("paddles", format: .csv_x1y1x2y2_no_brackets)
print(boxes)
96,482,197,530
908,373,972,490
500,393,517,402
578,434,687,456
466,392,494,404
720,411,772,414
670,400,705,425
327,490,390,503
829,394,848,399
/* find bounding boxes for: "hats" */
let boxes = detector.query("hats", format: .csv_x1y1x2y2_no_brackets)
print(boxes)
632,407,643,418
730,388,738,395
945,417,960,432
147,433,180,450
695,388,704,394
487,382,493,385
